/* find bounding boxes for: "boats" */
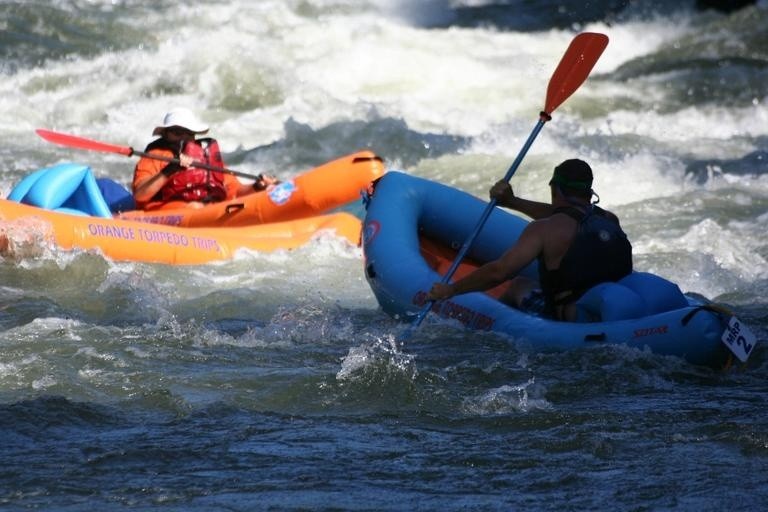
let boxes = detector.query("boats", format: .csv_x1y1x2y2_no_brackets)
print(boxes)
362,172,739,375
9,150,385,227
0,197,361,267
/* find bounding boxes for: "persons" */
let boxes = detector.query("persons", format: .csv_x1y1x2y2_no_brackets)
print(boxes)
130,109,277,210
427,158,631,322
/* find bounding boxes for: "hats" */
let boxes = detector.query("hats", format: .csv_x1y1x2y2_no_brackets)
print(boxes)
153,109,209,137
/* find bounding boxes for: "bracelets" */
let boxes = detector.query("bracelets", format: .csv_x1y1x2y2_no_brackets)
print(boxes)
252,182,262,192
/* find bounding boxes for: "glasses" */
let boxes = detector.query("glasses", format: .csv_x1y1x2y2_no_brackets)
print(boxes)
171,127,196,137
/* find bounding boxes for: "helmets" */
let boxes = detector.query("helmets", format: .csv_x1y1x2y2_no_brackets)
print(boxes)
549,157,594,200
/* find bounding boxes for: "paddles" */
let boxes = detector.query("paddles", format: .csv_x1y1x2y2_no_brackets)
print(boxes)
35,126,278,185
391,31,609,345
